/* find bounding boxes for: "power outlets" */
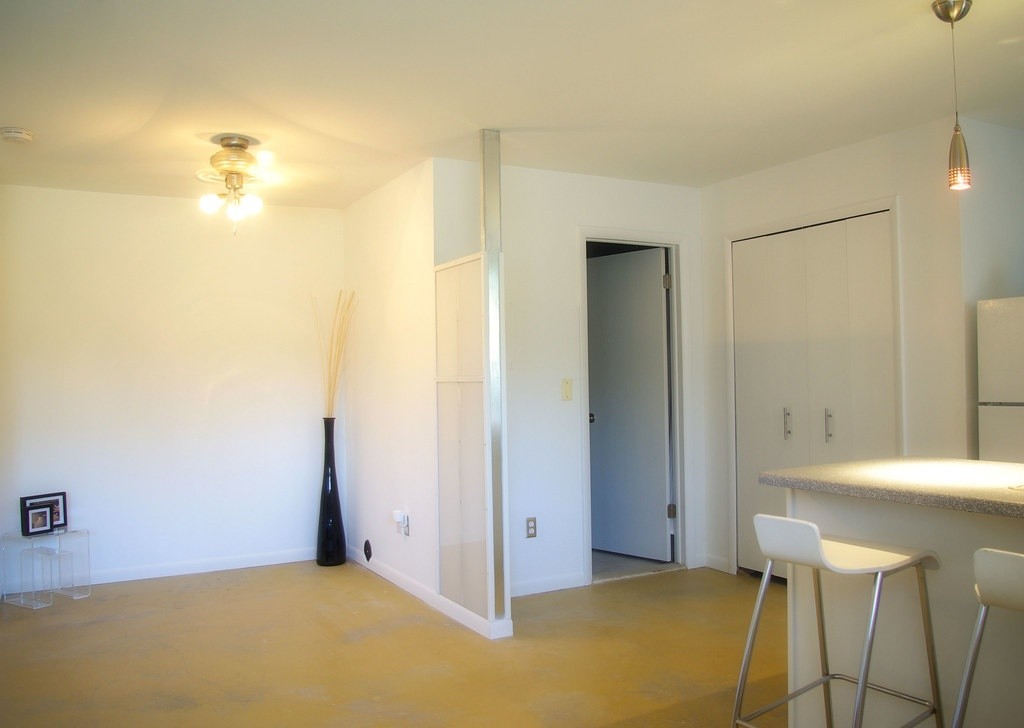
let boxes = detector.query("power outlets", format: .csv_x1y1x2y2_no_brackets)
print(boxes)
526,517,536,538
396,515,409,536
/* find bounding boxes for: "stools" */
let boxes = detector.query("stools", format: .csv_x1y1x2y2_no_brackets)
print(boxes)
952,546,1024,728
731,513,944,728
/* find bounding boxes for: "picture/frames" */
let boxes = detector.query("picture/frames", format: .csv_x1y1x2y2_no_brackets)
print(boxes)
20,491,68,532
24,503,54,535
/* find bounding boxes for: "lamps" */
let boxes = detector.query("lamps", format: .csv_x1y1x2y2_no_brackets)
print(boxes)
930,0,973,192
193,133,275,220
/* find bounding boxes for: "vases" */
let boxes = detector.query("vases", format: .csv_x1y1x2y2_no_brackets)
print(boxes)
316,417,347,567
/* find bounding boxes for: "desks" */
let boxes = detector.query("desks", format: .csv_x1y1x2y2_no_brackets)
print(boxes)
758,452,1024,728
